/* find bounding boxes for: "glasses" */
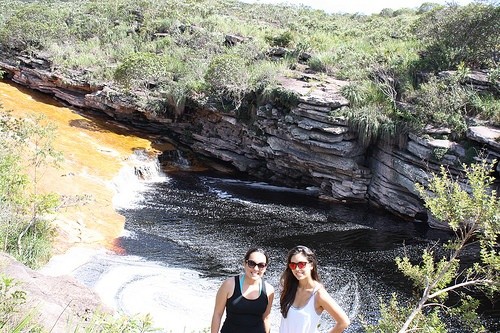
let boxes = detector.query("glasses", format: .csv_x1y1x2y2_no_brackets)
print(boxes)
285,262,311,270
245,260,267,269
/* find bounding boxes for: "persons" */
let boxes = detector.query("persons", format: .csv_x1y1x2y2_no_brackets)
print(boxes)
211,247,274,333
278,246,351,333
